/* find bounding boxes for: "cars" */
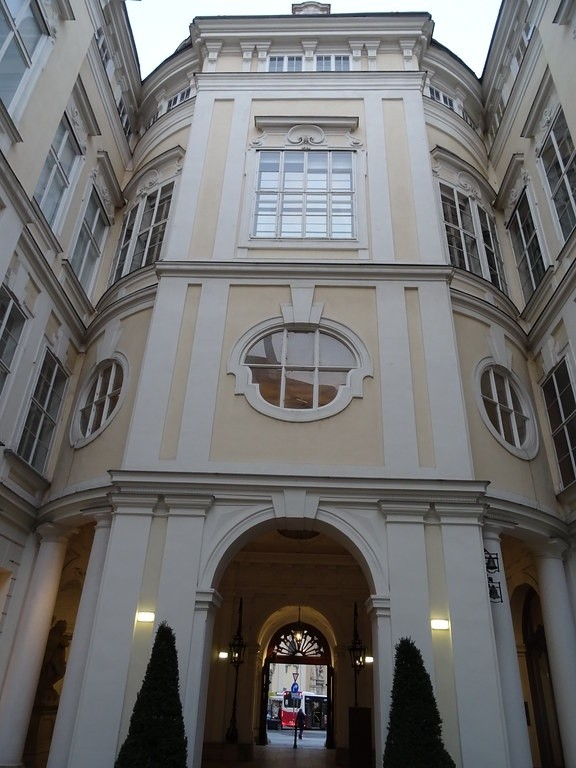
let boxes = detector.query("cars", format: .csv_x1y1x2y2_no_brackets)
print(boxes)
266,709,283,731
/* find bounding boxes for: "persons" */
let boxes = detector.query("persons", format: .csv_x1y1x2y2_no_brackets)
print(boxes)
296,709,305,739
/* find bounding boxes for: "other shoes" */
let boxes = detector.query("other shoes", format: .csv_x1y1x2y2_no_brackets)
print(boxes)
299,734,302,739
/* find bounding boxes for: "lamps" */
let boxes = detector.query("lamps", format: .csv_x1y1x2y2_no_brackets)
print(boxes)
347,638,368,673
294,604,303,645
227,635,248,669
484,548,503,603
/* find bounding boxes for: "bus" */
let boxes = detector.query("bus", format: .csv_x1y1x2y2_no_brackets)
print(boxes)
267,695,283,717
280,690,329,730
